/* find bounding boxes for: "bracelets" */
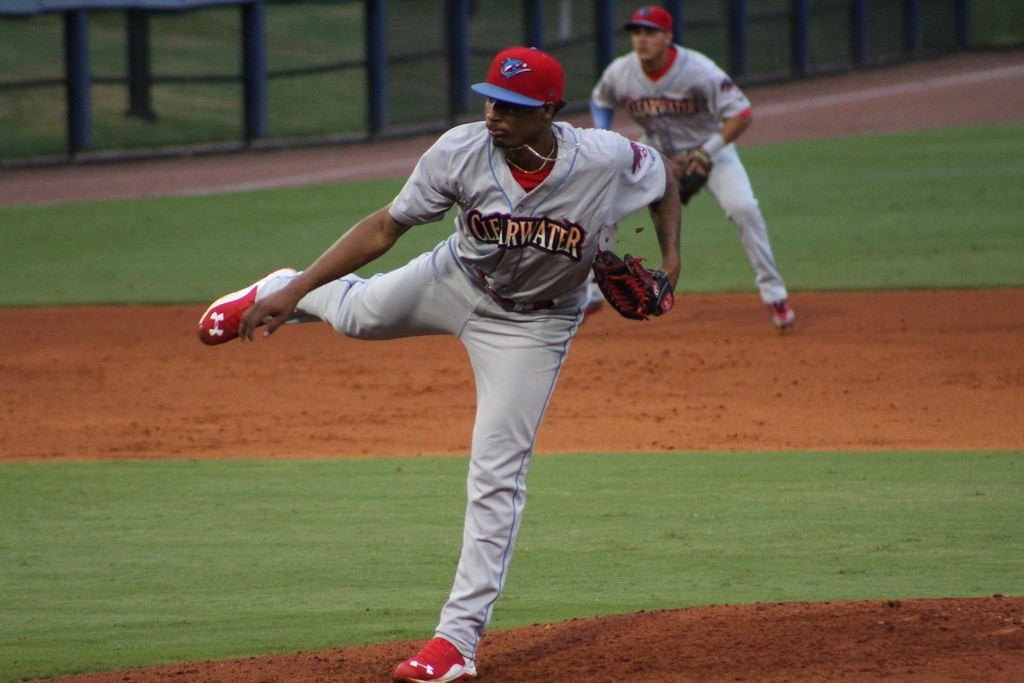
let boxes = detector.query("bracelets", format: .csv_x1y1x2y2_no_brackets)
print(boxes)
702,132,726,156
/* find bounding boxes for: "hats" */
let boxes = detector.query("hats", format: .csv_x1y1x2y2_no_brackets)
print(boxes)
471,47,564,106
624,6,672,34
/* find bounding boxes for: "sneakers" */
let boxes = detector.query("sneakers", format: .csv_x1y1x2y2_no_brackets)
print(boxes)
198,268,296,346
769,300,794,327
583,301,603,314
392,637,479,683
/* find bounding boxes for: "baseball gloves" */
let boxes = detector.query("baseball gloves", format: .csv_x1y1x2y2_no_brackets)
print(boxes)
589,249,675,321
668,147,715,207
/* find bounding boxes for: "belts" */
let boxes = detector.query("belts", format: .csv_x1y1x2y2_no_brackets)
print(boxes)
475,270,552,311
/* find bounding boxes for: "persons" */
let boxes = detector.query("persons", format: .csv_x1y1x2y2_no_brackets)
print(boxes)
197,47,682,683
590,5,795,327
